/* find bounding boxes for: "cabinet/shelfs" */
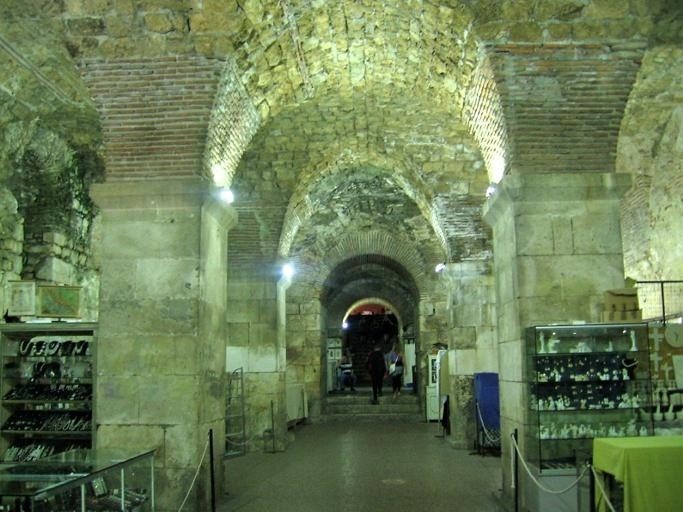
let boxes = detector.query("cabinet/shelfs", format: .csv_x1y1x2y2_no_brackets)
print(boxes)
0,323,97,465
525,322,652,511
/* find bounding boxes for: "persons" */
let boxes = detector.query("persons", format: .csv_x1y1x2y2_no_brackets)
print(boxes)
332,346,359,392
386,341,406,400
366,342,386,405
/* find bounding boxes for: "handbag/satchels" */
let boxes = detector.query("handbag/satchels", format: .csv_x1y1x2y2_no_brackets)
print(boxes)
388,364,396,375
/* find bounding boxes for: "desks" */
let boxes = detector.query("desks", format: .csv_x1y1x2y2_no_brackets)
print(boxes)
590,434,682,511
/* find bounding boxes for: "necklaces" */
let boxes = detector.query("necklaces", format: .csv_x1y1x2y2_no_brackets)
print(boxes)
3,340,87,461
620,358,640,368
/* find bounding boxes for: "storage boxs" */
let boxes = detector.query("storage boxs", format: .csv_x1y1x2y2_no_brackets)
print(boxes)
599,288,642,322
